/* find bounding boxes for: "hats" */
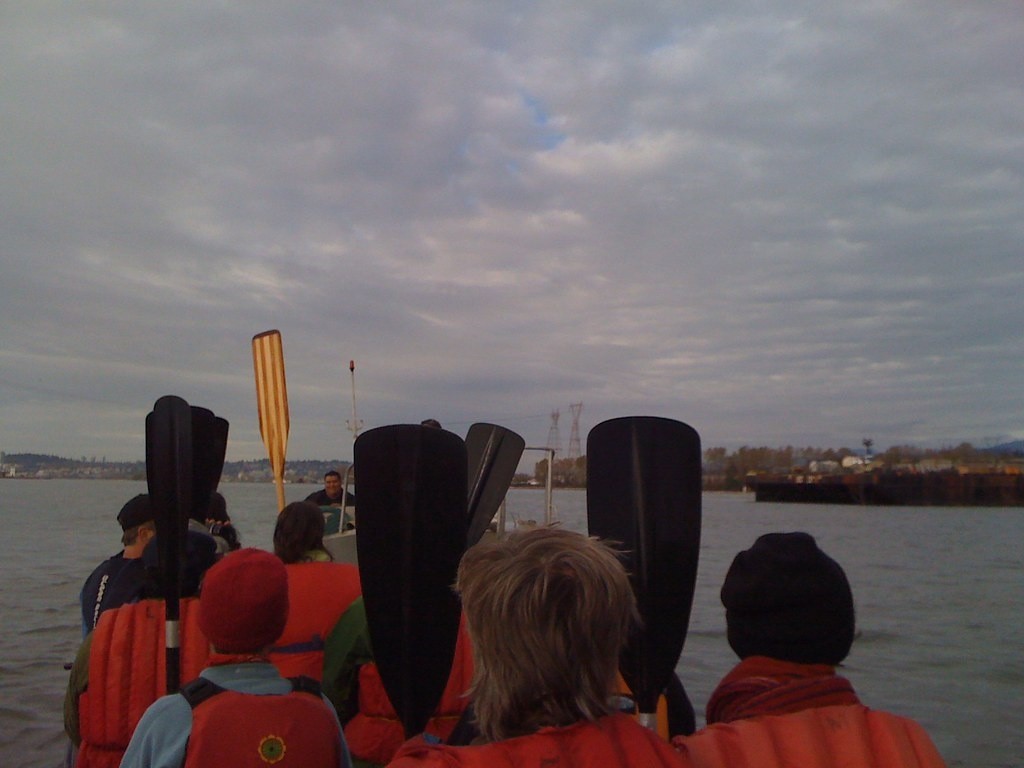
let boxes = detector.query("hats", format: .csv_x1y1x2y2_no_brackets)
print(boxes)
198,548,290,652
719,534,854,667
116,494,155,531
144,530,216,572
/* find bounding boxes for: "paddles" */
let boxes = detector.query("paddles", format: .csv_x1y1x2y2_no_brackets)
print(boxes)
464,423,525,549
252,329,290,515
352,423,468,742
586,416,703,733
145,395,229,541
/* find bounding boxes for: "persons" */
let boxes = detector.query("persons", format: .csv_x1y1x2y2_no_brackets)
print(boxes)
102,547,353,768
79,489,242,640
669,530,952,768
266,499,360,694
64,529,217,768
302,471,357,507
378,529,703,768
419,420,441,429
